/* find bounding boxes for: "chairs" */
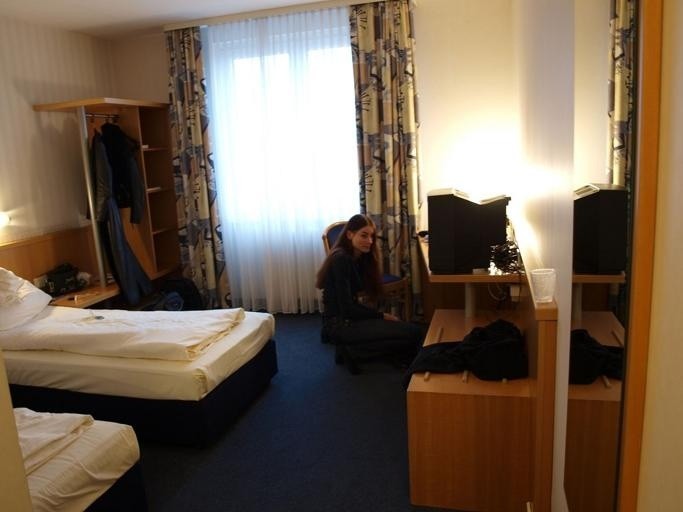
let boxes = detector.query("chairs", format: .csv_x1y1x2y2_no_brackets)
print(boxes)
321,220,411,325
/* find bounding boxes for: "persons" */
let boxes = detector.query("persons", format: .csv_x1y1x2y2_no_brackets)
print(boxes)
312,213,423,369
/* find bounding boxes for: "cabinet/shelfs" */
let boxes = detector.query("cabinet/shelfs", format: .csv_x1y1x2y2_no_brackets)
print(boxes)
31,97,184,310
563,305,626,511
405,225,535,512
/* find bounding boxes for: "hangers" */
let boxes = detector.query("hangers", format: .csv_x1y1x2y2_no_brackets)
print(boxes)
88,113,125,148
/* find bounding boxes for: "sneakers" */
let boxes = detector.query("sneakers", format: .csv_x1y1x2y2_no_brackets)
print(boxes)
334,344,371,376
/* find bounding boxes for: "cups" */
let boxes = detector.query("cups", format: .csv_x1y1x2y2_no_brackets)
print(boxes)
530,268,557,304
43,277,57,298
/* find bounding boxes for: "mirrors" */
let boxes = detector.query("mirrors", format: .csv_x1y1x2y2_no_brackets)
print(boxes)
558,0,664,512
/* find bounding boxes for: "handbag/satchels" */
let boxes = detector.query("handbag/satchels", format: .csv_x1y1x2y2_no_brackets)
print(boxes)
489,243,521,272
46,262,81,297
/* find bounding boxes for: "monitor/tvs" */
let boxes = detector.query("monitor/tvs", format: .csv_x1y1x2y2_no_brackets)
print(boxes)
427,188,511,274
573,183,627,275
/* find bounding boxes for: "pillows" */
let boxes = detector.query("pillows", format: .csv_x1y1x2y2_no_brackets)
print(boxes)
0,264,52,332
